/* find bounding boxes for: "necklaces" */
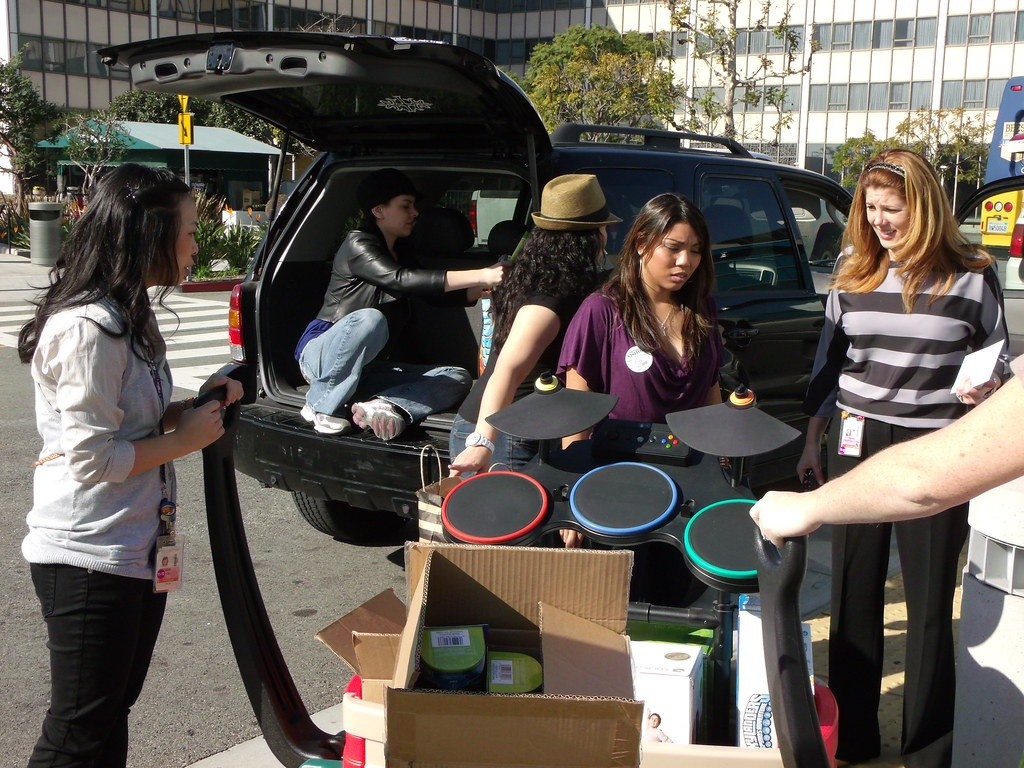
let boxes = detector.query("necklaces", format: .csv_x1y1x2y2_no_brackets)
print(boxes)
653,300,673,336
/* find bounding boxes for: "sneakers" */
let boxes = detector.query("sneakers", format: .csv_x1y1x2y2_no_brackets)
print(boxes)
351,398,406,441
300,404,351,435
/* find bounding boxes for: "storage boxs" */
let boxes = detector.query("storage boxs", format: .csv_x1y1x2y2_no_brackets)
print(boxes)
314,537,645,765
628,639,706,741
736,593,817,747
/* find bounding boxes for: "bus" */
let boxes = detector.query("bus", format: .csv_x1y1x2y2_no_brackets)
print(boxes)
980,76,1024,260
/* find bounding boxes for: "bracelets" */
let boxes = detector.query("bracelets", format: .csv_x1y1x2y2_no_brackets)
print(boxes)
465,432,495,455
180,397,197,410
984,378,997,399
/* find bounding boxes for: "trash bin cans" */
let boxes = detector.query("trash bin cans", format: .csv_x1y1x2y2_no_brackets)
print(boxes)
28,202,64,265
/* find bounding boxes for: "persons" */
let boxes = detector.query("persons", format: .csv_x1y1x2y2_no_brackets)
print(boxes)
749,354,1024,549
293,168,502,436
555,192,732,605
447,174,623,478
21,162,245,768
796,147,1015,768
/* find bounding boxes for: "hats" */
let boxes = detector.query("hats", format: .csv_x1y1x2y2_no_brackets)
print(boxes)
357,168,417,213
530,174,624,231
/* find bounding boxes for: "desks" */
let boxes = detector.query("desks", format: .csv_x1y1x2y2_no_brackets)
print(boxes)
186,701,784,766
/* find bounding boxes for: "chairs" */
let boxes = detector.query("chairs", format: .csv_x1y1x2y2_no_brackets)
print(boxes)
697,204,751,298
388,206,530,372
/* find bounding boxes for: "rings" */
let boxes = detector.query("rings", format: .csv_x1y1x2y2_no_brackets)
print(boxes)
957,394,961,398
763,535,767,541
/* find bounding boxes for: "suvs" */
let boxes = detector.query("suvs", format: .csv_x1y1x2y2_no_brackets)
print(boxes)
97,32,1024,541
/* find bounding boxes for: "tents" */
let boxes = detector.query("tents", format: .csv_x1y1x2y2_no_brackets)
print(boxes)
33,119,297,210
